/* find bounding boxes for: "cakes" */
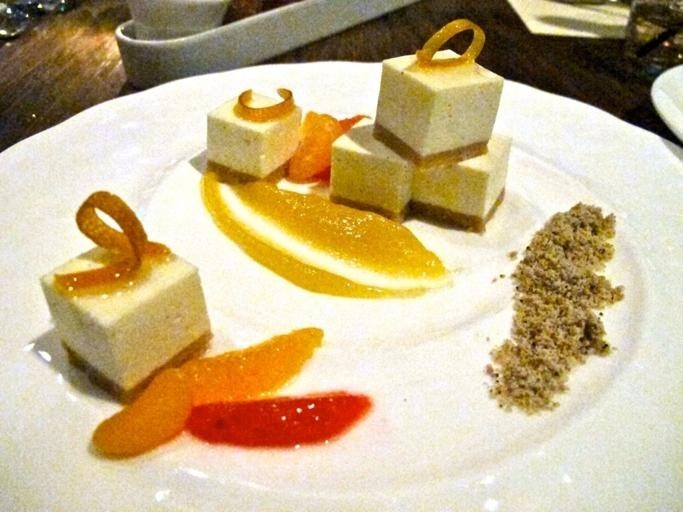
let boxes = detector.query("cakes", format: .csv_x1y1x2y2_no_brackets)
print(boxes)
39,233,213,406
409,134,513,236
329,116,413,225
207,89,303,186
371,49,504,171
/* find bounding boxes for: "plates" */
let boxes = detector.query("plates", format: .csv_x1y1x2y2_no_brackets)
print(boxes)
0,60,683,512
649,63,683,143
